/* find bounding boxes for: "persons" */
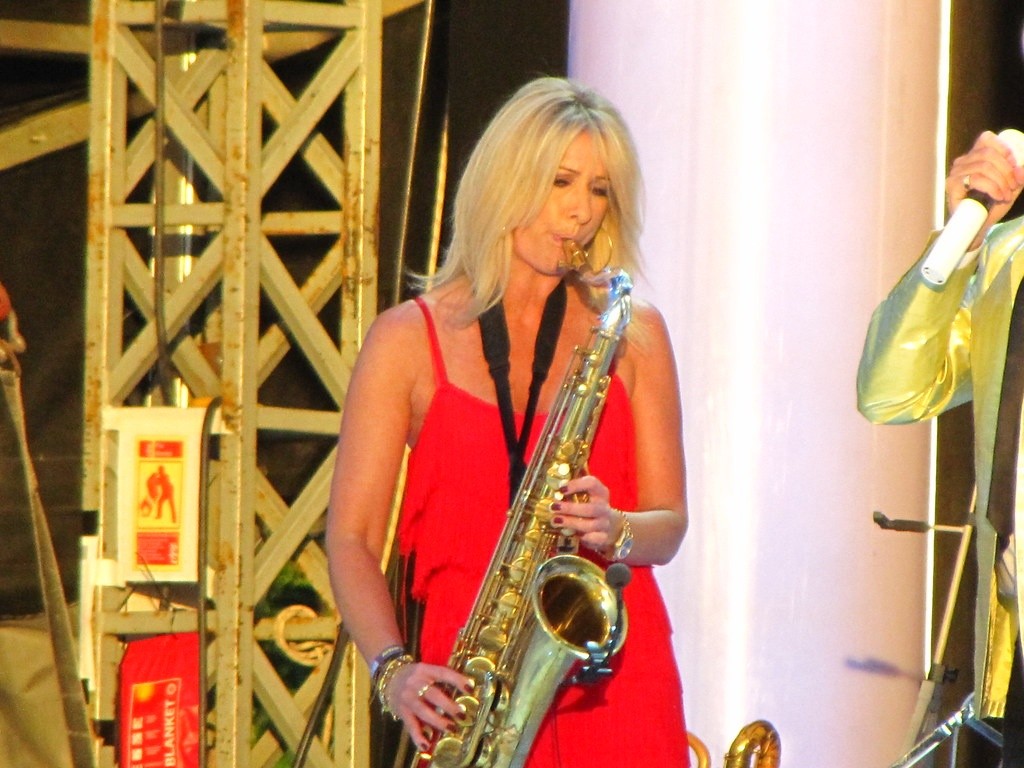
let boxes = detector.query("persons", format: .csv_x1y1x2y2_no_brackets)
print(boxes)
326,78,691,768
857,130,1023,768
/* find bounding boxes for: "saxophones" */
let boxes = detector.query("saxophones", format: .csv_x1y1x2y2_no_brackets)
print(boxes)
411,237,633,768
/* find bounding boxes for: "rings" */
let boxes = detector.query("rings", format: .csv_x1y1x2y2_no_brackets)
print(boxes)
963,175,971,191
418,682,433,697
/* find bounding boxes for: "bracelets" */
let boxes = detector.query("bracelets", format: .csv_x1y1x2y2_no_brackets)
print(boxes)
370,645,414,720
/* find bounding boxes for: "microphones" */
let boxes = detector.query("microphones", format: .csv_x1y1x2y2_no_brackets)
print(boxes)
607,564,631,589
921,129,1024,285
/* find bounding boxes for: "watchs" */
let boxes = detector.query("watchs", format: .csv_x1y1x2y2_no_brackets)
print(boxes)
594,507,634,563
